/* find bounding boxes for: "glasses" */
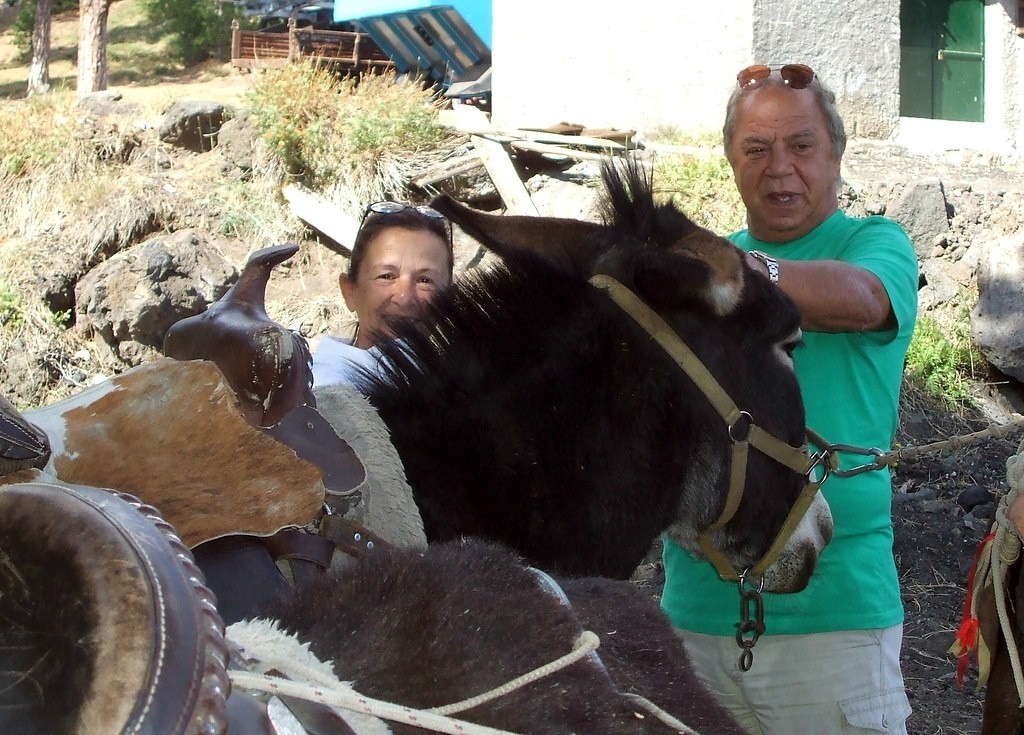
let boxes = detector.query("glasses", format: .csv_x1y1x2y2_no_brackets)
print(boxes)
352,201,454,259
737,63,823,91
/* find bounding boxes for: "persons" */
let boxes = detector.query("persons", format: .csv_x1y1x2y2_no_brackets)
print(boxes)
327,206,455,363
654,68,919,735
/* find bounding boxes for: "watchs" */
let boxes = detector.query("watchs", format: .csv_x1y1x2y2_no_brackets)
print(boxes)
747,250,778,285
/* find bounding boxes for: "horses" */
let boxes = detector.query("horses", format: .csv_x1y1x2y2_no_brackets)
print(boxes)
0,138,1024,734
